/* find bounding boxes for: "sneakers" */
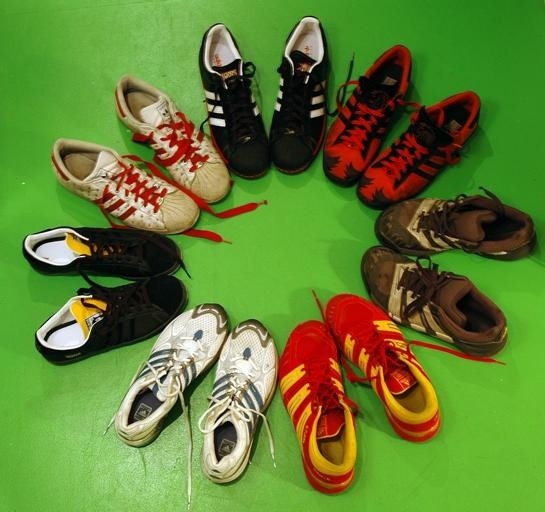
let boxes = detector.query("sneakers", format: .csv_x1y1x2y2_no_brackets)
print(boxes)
278,320,359,494
322,295,441,442
361,246,508,357
199,24,272,179
35,273,188,366
52,138,200,235
268,16,331,174
198,319,278,483
103,304,228,447
22,227,181,280
323,45,422,187
115,76,232,204
375,195,536,261
356,92,480,210
